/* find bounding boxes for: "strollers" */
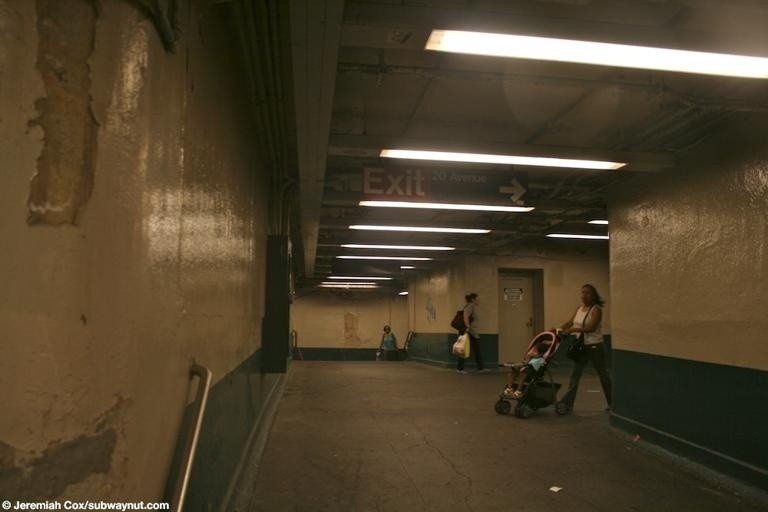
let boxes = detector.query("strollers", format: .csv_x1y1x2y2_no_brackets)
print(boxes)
489,326,585,420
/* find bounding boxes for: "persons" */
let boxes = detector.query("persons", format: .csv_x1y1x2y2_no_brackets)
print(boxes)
378,325,399,361
504,284,612,415
456,292,492,374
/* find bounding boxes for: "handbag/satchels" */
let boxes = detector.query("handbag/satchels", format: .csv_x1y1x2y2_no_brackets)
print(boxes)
450,304,473,331
376,352,382,361
394,349,407,361
566,333,585,360
452,332,470,358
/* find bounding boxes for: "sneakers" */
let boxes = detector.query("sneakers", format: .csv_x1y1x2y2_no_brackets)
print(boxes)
457,369,468,374
503,389,524,398
479,369,491,374
